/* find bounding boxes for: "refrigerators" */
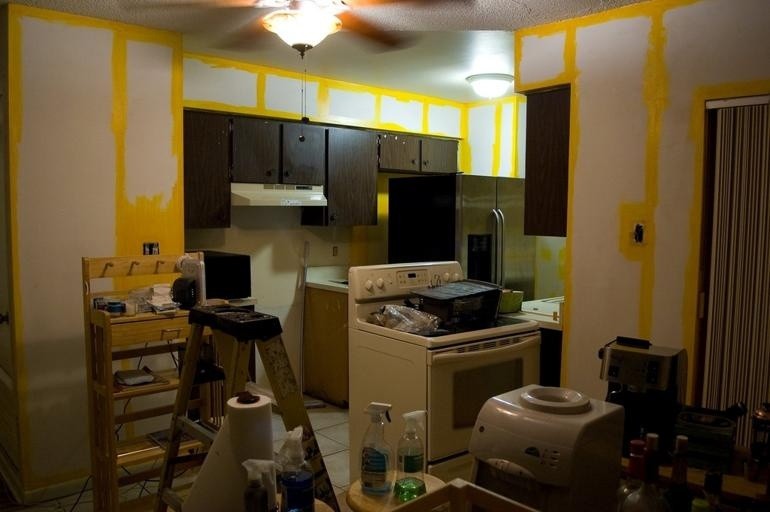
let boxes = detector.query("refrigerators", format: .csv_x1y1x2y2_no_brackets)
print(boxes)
386,172,537,301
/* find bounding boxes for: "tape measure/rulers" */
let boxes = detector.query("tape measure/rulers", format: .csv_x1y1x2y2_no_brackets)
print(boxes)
106,301,126,312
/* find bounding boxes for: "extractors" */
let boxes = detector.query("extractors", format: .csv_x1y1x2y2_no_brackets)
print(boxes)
231,182,328,209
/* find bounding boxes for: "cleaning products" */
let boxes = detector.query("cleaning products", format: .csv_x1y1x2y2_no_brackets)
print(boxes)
396,410,428,501
281,432,314,512
243,461,270,512
357,403,393,494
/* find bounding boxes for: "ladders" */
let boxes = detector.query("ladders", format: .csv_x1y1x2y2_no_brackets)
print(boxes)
153,302,342,512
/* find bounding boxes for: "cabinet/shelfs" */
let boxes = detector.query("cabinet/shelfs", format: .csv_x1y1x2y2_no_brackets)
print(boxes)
184,109,231,227
83,254,210,510
524,89,569,237
381,134,457,174
301,128,379,227
304,288,349,408
232,116,327,187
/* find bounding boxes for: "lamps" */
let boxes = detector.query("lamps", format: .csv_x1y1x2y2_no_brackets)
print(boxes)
260,9,343,142
465,73,517,102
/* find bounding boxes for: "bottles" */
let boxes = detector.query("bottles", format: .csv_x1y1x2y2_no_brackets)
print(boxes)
618,431,724,512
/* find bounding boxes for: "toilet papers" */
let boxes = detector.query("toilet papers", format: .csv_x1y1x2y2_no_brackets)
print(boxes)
183,396,274,512
